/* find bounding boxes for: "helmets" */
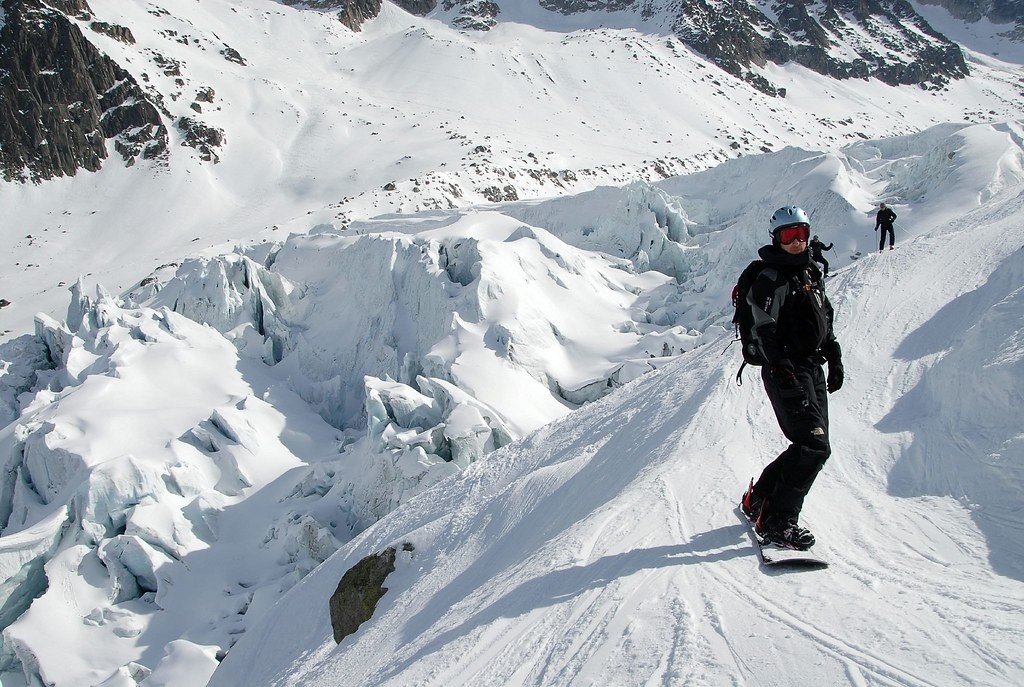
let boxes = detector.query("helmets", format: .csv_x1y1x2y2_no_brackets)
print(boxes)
768,205,811,239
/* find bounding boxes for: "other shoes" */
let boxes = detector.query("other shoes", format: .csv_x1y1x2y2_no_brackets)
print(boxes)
890,246,894,249
824,274,829,278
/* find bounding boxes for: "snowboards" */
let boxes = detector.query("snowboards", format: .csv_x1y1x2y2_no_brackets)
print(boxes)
738,500,830,568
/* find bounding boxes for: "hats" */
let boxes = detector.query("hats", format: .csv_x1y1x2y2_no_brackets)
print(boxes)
813,235,818,239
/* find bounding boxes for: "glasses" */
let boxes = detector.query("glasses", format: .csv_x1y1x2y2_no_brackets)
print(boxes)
768,224,810,243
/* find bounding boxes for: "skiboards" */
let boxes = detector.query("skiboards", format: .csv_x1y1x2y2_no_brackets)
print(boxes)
879,247,899,253
829,272,839,279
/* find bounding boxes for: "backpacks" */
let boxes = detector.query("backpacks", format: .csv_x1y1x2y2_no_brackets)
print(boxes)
734,258,792,386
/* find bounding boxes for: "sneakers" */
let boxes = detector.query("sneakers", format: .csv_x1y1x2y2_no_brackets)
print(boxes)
754,511,816,550
739,477,763,522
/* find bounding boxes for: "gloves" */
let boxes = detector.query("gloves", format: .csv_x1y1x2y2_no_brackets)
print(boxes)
875,227,878,232
820,341,844,393
773,358,808,415
830,242,834,247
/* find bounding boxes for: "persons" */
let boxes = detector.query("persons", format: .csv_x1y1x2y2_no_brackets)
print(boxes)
808,235,833,279
874,203,897,253
742,207,845,551
